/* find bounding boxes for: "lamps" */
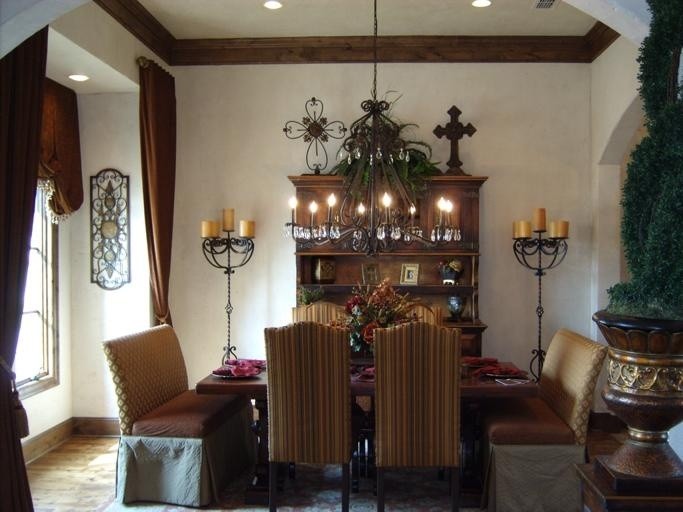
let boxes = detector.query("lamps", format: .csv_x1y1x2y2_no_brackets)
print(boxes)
285,0,461,253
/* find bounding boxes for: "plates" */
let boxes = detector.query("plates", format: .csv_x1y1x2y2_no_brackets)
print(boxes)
460,362,495,368
211,366,258,378
485,371,517,378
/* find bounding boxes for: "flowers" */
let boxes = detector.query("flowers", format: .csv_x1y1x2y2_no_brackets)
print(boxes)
436,260,464,272
329,279,435,351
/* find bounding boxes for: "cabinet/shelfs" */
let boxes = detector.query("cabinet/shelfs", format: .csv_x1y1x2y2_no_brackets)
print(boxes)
575,463,683,512
287,175,487,357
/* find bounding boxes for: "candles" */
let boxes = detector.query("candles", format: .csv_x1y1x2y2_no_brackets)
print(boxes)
511,207,569,240
202,206,255,240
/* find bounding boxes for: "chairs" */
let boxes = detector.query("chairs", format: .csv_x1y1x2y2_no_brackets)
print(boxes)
372,321,462,511
263,322,358,512
480,327,608,512
290,301,366,475
389,301,442,325
102,324,258,506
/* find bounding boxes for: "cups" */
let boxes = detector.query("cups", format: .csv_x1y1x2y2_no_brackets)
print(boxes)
446,292,466,318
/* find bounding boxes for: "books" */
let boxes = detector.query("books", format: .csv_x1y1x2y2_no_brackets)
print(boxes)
512,378,529,384
495,378,521,385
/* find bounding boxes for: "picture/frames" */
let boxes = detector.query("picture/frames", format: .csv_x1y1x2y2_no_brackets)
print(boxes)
399,263,420,285
360,263,381,285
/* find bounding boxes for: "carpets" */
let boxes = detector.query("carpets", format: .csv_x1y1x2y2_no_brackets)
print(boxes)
103,462,481,511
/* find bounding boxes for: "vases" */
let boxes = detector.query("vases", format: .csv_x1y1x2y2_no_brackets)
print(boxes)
441,267,459,285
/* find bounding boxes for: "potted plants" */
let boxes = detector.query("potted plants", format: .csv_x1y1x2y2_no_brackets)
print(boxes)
590,1,683,476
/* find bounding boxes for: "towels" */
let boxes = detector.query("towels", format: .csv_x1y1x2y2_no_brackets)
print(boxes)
365,367,375,374
462,355,498,366
212,366,262,377
471,366,528,381
